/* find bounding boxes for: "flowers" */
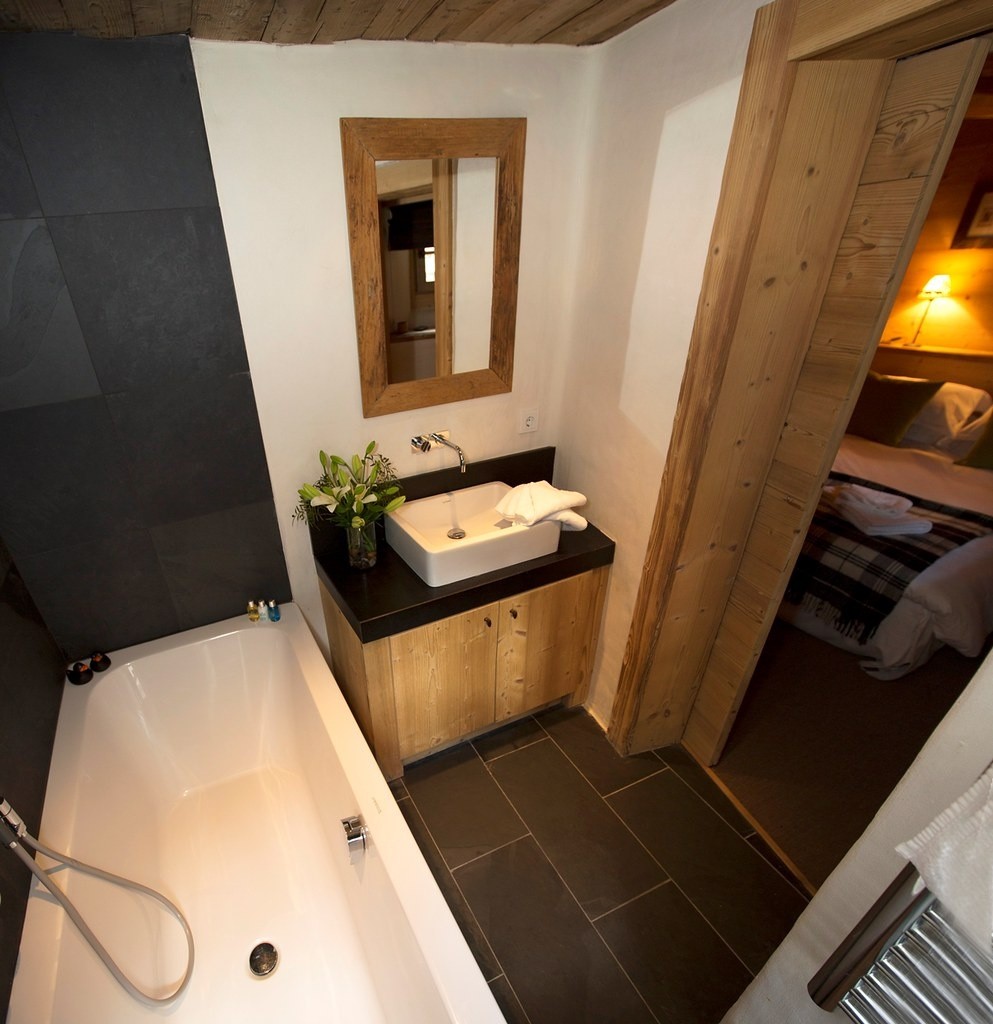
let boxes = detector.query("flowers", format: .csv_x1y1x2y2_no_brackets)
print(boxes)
291,440,406,530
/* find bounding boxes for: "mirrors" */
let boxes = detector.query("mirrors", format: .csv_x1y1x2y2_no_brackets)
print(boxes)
339,115,527,419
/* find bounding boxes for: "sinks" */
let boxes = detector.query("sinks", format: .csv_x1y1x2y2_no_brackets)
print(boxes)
384,480,562,587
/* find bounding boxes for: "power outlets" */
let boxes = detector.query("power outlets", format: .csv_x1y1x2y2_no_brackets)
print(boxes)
520,410,540,433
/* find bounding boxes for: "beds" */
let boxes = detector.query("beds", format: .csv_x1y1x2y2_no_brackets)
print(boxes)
777,342,993,681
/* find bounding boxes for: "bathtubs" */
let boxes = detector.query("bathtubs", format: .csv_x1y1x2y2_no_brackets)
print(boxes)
7,600,502,1022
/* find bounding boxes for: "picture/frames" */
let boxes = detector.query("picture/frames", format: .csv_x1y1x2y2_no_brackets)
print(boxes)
950,184,993,249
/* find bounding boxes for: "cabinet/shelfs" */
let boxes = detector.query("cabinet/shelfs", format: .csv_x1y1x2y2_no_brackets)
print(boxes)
317,564,611,782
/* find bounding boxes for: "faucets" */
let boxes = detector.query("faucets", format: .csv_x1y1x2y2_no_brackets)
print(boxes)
431,433,467,474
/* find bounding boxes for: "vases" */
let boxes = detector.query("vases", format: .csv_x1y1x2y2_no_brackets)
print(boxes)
347,521,384,583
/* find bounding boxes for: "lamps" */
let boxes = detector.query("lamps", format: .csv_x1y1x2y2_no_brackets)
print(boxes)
906,274,950,347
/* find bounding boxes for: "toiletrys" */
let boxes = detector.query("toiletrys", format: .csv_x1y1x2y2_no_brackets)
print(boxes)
246,599,281,623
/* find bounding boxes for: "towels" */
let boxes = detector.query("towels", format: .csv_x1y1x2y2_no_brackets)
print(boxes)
820,482,929,538
493,478,587,533
891,756,989,973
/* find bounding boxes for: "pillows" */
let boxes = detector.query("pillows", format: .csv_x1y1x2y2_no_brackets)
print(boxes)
845,370,947,448
886,375,990,453
